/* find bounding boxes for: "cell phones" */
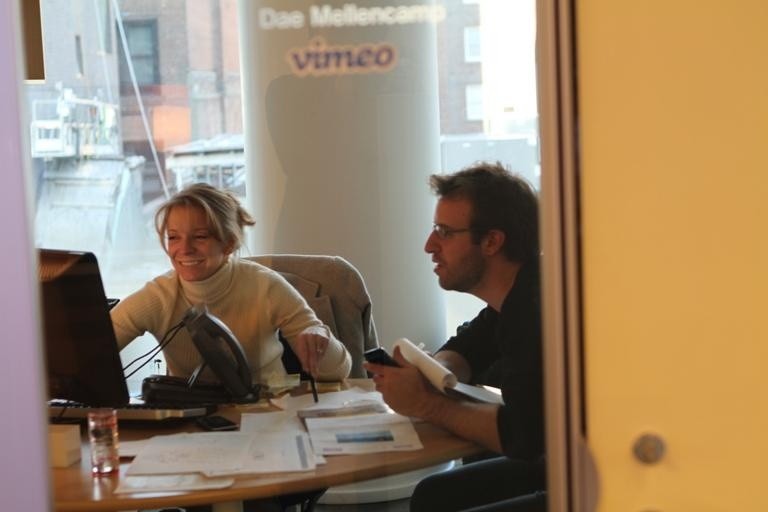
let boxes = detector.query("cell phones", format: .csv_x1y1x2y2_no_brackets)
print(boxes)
198,415,240,432
364,346,402,369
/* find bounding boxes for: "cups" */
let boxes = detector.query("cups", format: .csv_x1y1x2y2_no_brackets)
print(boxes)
88,407,121,476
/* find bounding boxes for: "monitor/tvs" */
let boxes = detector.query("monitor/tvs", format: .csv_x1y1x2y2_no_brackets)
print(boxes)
36,248,131,409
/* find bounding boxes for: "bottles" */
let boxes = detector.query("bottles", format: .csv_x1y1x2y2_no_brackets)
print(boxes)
109,428,111,430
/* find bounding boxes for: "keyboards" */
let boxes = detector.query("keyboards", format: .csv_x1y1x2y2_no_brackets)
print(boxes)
49,402,207,427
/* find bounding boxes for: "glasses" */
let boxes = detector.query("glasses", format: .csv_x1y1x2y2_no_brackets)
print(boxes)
433,223,471,236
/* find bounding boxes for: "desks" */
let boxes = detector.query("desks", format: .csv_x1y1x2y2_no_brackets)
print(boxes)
49,378,504,511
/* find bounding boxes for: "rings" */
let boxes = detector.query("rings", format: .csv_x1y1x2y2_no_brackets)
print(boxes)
317,348,323,354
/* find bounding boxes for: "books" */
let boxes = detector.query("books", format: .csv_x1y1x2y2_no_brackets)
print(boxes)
395,337,504,407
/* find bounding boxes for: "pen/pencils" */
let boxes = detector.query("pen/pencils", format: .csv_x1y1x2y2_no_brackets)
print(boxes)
310,375,319,402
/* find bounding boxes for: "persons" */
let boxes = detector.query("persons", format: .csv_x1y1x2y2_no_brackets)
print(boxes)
108,182,352,382
363,160,546,512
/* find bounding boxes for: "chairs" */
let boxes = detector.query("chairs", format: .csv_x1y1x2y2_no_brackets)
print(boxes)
243,254,379,377
